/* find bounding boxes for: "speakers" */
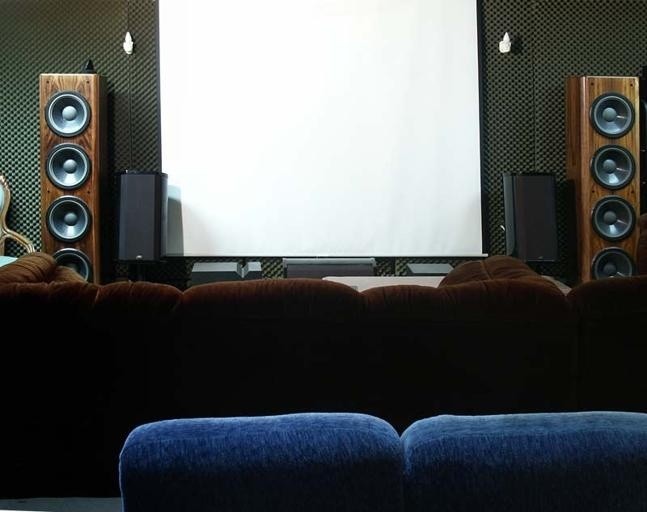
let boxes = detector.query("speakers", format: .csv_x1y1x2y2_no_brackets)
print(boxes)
118,174,162,261
502,173,559,263
284,258,377,278
40,73,109,286
566,76,640,284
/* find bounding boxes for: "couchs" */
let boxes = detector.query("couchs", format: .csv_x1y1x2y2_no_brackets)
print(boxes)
0,254,647,497
119,411,647,512
